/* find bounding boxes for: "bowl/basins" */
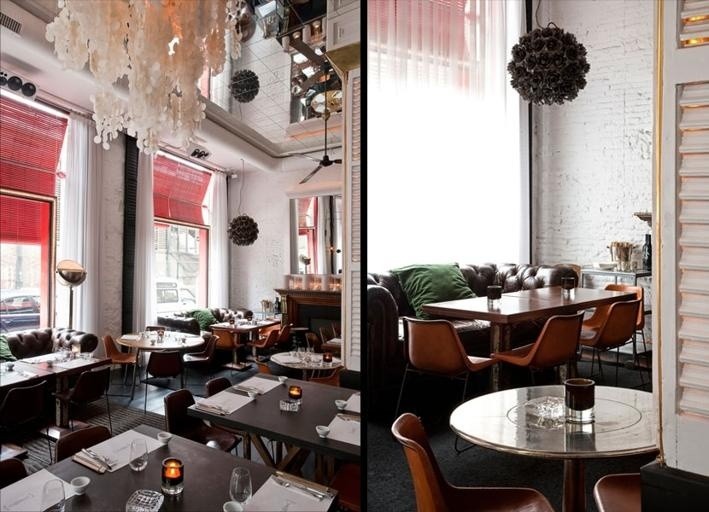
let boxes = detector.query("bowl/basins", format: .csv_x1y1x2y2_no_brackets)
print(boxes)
278,376,288,383
156,431,174,444
335,399,347,410
223,501,244,511
71,477,92,496
247,391,258,399
315,425,330,439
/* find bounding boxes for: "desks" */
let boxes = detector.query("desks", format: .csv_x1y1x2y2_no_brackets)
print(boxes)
114,329,206,415
449,384,656,512
269,351,342,372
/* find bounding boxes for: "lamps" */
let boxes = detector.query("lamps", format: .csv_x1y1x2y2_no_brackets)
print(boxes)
57,259,87,328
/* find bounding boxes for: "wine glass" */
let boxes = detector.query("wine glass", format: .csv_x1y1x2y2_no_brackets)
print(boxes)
228,467,253,506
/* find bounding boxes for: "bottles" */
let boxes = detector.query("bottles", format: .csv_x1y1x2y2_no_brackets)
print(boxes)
640,234,653,271
268,302,273,312
274,296,281,315
262,300,267,313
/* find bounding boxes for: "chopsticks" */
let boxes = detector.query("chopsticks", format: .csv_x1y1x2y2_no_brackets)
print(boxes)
75,453,101,470
197,402,224,412
282,475,330,493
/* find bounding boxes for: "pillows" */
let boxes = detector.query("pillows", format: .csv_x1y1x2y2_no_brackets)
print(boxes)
389,263,478,321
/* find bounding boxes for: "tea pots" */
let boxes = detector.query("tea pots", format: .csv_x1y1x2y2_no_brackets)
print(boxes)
606,241,632,264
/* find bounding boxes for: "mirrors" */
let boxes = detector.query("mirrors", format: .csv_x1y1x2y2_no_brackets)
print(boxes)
290,194,343,275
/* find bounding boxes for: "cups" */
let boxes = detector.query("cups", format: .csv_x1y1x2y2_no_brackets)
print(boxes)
41,478,66,512
561,277,576,289
615,253,640,271
486,285,502,300
7,363,14,371
487,299,501,312
323,353,332,367
288,385,303,406
139,327,186,345
47,360,52,368
65,351,93,363
562,287,575,302
288,346,315,365
160,456,186,496
251,320,258,325
562,378,597,425
129,438,149,472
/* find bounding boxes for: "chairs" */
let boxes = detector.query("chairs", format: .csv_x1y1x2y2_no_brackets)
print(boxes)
489,311,586,386
582,298,645,387
0,459,28,490
393,316,492,454
390,413,555,512
592,473,642,512
582,284,648,366
0,308,341,462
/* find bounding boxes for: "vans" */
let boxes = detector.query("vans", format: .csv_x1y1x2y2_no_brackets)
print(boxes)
155,280,198,312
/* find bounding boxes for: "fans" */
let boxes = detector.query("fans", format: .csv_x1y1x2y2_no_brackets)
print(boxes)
287,64,342,184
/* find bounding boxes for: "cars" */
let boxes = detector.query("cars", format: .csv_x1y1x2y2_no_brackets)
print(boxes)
0,292,40,331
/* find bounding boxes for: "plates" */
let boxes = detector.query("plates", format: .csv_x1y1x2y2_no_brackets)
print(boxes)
599,263,616,270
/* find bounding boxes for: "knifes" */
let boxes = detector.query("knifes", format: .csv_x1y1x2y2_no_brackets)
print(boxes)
272,476,324,500
195,407,225,416
81,447,112,471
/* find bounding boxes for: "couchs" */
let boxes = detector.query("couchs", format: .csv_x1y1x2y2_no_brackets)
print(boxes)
367,263,581,416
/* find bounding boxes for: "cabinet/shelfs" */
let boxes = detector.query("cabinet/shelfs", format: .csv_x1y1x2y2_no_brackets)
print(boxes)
580,268,652,363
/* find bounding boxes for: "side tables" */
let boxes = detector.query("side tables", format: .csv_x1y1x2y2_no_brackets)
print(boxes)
0,348,112,403
188,370,361,450
210,316,281,333
